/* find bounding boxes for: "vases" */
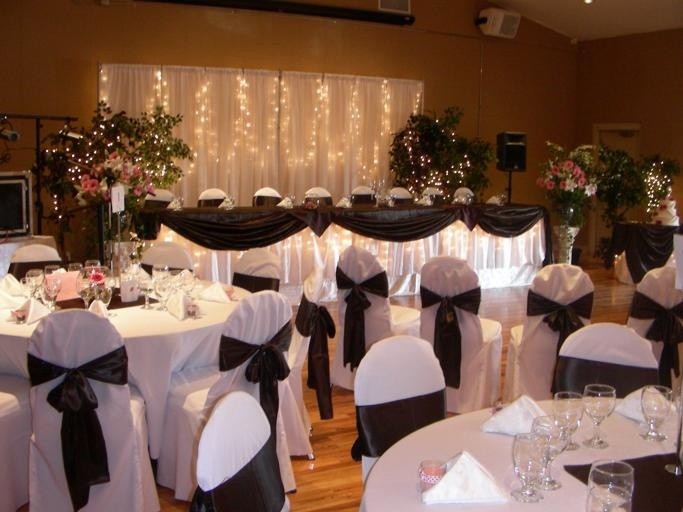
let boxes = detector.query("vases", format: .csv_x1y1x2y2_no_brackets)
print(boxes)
550,224,581,267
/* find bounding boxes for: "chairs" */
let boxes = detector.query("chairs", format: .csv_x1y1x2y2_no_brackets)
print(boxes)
452,187,475,204
196,187,228,208
421,187,443,205
250,186,282,208
388,186,413,205
348,185,377,207
304,186,333,207
142,190,176,209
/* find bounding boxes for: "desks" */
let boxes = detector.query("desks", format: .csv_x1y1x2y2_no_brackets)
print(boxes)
132,203,553,304
610,222,683,284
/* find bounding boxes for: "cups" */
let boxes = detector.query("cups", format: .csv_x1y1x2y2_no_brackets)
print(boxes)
419,459,447,494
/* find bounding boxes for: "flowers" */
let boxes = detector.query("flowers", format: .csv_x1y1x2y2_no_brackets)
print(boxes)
80,149,155,236
531,137,605,230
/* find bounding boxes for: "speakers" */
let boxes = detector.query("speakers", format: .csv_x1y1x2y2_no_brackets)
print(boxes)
497,131,527,172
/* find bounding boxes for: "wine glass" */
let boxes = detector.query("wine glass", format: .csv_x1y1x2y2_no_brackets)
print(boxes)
23,259,170,314
511,382,674,511
